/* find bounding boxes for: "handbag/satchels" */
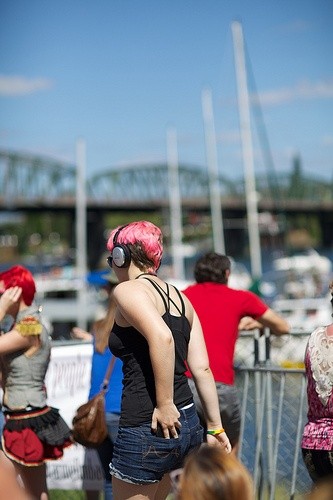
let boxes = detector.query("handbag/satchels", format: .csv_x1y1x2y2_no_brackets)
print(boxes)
71,357,116,445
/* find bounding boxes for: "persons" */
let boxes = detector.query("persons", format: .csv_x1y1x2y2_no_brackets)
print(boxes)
175,252,288,458
0,264,77,500
300,282,333,499
87,262,126,500
106,221,232,500
130,443,254,500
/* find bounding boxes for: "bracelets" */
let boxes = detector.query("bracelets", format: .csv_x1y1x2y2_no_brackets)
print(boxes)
206,429,226,435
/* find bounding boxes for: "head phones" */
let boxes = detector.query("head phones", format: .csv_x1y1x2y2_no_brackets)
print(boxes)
111,224,131,268
